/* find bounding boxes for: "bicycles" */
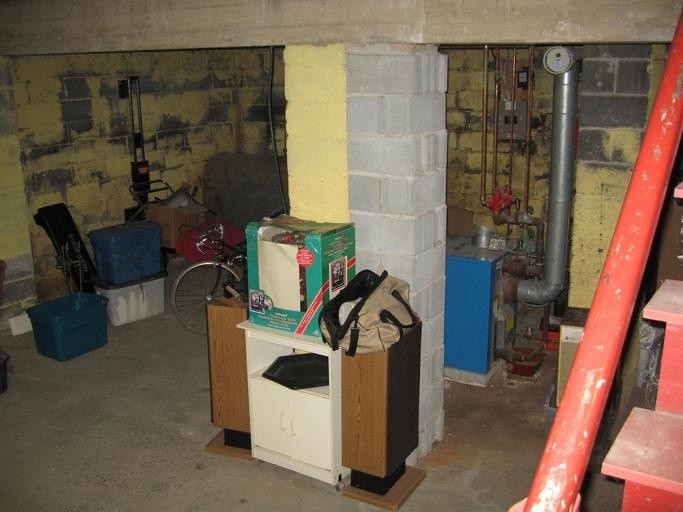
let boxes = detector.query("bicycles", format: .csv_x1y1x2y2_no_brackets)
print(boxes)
169,207,286,335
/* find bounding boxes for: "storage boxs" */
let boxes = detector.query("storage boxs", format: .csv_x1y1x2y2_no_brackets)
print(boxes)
25,201,208,363
244,213,357,347
543,307,624,454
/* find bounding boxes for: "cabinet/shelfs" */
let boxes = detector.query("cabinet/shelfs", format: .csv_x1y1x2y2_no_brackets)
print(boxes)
236,319,351,488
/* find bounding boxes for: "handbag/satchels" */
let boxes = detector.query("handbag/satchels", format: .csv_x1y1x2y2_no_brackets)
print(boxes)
319,270,420,356
